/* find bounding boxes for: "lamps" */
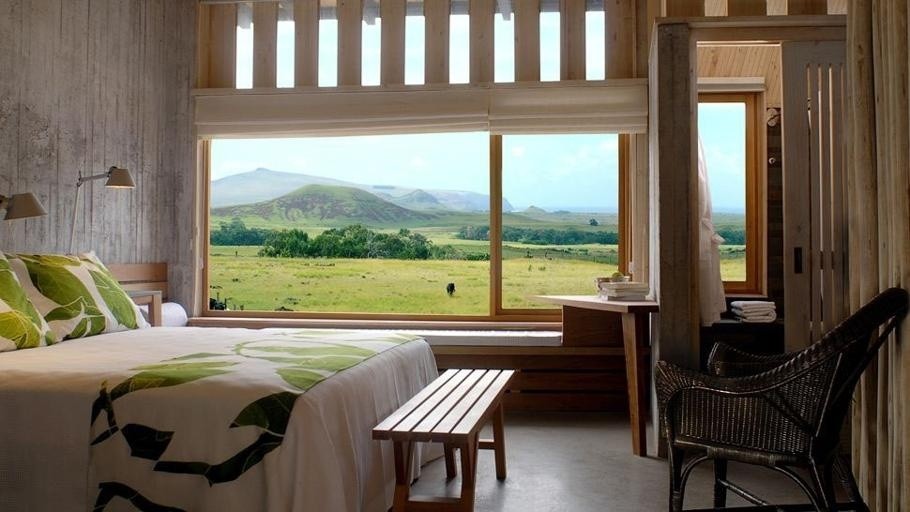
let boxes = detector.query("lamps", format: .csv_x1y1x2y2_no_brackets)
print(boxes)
0,190,49,221
67,165,136,255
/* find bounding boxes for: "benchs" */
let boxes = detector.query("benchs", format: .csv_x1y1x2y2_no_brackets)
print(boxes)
371,366,518,512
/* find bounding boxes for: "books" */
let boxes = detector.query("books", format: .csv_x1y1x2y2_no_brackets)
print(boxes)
599,280,650,302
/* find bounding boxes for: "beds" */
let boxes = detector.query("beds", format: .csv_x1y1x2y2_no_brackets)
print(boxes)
0,261,449,511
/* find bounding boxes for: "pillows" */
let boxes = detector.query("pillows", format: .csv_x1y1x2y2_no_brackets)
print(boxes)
0,251,148,354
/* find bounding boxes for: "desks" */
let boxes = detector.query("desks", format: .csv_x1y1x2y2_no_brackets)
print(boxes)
528,291,660,460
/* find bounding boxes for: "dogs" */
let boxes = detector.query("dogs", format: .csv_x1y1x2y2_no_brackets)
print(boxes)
446,282,454,295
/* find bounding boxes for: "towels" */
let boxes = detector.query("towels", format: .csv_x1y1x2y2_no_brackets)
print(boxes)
730,299,779,324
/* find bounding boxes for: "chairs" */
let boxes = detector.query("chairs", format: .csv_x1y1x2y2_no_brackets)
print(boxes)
650,285,910,511
